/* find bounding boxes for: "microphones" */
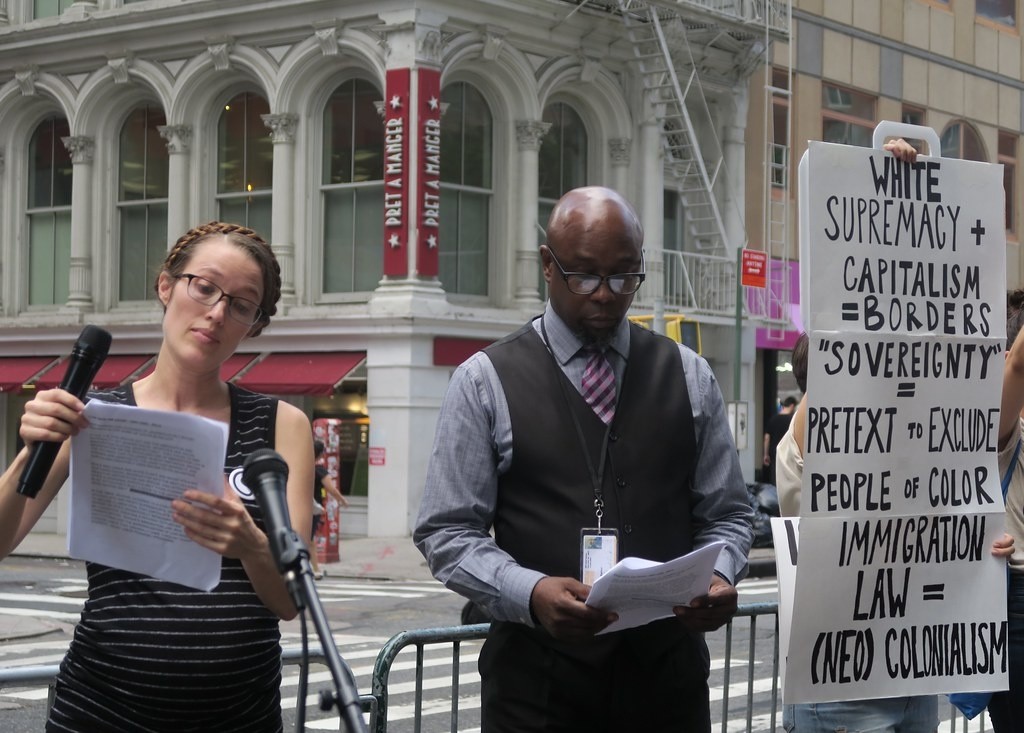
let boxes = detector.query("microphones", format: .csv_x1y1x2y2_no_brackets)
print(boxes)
17,325,112,500
241,449,306,612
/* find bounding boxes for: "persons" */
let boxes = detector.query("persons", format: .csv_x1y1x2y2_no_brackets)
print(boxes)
764,137,1024,733
306,440,352,579
411,184,758,733
0,222,315,733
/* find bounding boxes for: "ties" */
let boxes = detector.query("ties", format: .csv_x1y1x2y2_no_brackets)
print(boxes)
581,340,617,426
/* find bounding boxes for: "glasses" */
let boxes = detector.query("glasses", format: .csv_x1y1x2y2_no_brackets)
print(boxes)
547,245,644,296
174,273,265,325
